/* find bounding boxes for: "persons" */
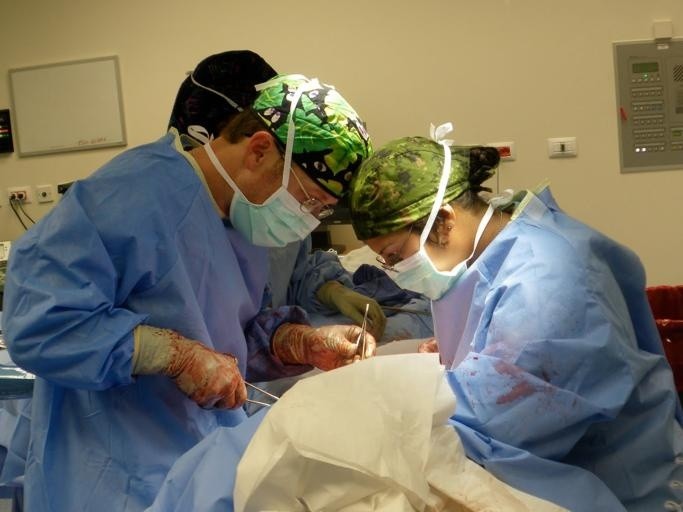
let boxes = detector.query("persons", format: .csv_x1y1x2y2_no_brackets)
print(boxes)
0,70,379,512
347,133,682,512
262,230,387,345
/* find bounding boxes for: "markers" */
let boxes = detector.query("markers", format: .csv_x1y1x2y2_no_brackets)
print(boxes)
0,118,9,138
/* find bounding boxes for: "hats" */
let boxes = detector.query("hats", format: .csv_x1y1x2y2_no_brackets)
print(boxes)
252,75,371,199
168,52,279,146
352,137,472,240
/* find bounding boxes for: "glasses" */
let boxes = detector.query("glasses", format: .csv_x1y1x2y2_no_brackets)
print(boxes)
375,222,415,274
245,131,334,220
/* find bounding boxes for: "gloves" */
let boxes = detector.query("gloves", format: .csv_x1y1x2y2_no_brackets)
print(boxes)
130,323,248,409
315,280,386,342
272,324,374,372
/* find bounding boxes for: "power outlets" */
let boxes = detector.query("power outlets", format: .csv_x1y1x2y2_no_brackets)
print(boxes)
6,185,32,206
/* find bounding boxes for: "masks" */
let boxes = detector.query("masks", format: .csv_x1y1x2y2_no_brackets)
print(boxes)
378,194,494,301
206,128,320,248
